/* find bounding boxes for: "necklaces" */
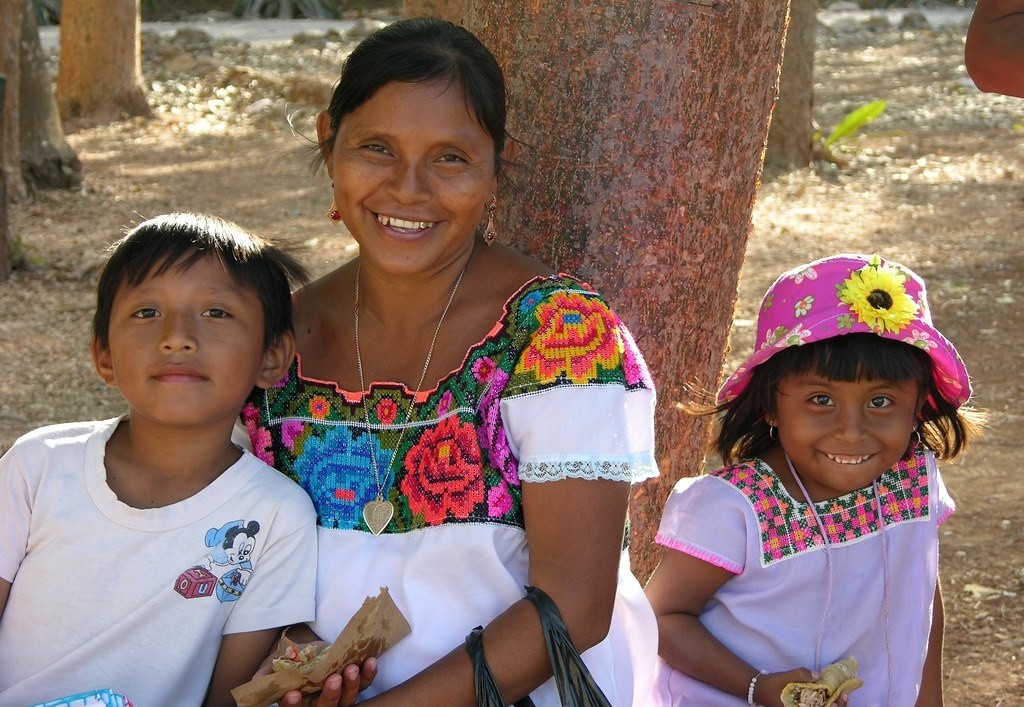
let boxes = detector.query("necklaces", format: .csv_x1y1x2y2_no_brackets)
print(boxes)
353,265,463,536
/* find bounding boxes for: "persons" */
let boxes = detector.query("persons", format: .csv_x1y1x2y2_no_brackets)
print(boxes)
233,15,660,707
0,211,318,707
641,255,989,707
964,0,1024,98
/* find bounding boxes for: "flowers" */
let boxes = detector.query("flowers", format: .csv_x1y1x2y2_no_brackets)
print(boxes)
839,264,921,335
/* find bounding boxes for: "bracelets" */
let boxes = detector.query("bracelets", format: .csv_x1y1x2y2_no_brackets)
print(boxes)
748,669,769,707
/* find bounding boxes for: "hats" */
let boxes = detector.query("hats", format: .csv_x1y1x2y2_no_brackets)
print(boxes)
716,255,973,422
